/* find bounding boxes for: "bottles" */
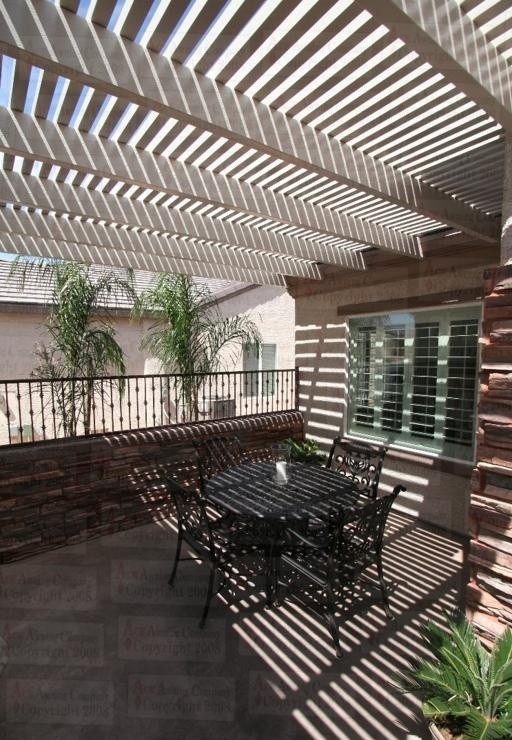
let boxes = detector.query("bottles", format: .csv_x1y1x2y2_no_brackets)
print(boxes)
272,443,291,485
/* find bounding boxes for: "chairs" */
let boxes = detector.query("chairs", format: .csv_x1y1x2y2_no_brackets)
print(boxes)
163,467,280,631
283,481,407,657
193,435,251,469
325,435,389,500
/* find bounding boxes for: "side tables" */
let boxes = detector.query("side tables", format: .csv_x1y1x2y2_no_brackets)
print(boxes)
201,457,360,557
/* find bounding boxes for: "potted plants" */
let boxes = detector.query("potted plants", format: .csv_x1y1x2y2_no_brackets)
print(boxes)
392,606,511,740
